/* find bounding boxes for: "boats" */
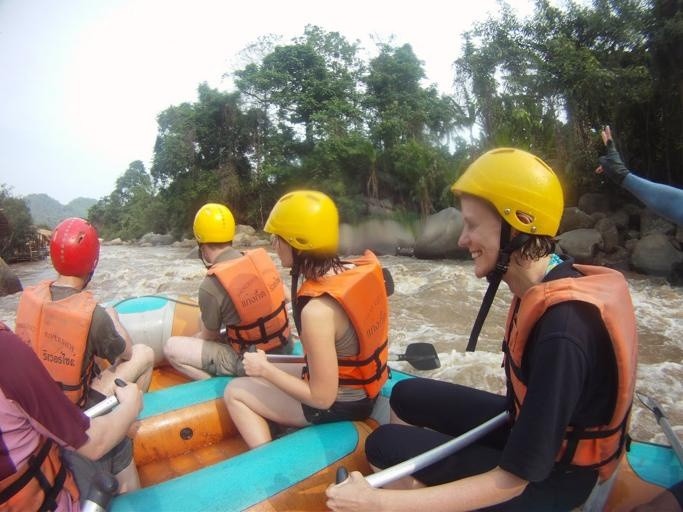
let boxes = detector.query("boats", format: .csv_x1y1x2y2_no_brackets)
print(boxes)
79,297,681,511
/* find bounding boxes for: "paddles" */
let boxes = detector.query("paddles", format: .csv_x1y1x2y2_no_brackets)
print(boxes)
638,391,683,469
250,342,441,372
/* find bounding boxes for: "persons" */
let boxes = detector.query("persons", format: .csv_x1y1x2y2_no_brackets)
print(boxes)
0,256,145,511
222,189,392,450
14,216,156,414
590,120,683,231
321,144,643,511
161,203,295,382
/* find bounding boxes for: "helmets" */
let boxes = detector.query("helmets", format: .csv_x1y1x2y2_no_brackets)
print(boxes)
49,218,99,278
192,203,235,243
449,146,563,238
262,190,338,252
